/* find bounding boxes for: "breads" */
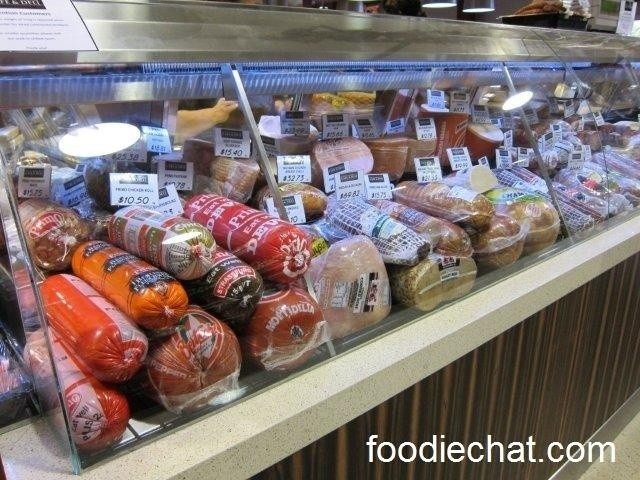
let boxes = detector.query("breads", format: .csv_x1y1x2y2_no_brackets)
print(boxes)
212,135,560,338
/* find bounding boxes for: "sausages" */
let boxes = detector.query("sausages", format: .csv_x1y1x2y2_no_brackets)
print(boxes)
18,193,323,452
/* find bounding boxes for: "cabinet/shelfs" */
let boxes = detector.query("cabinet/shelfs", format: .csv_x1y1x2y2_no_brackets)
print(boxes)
0,0,638,480
